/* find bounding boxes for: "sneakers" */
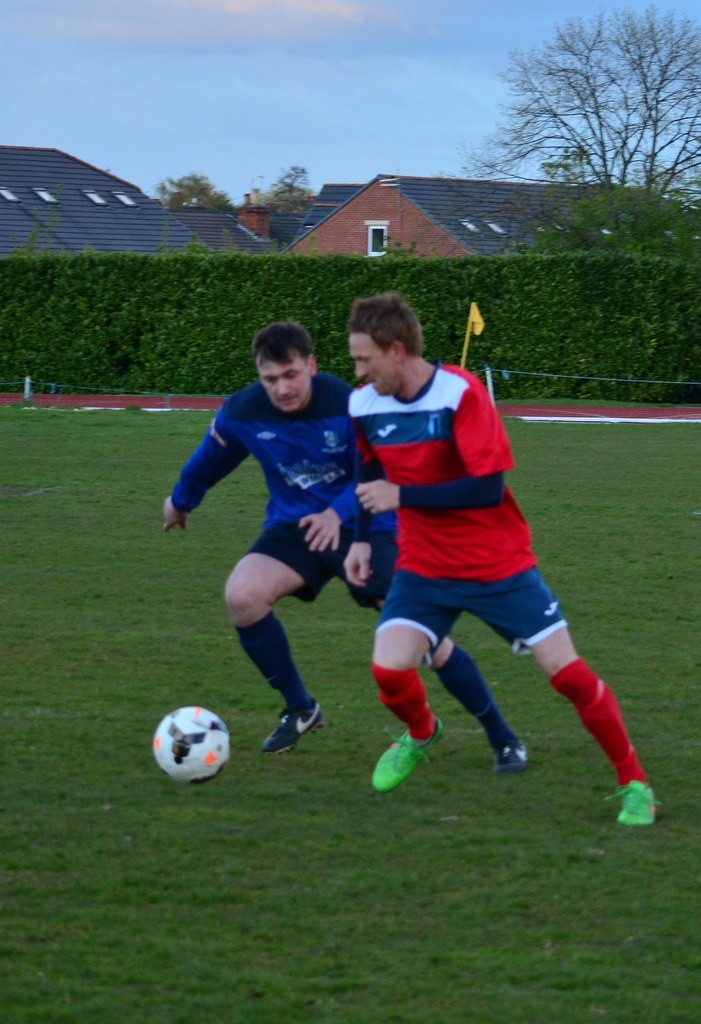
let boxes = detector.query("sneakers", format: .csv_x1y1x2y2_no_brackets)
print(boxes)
262,698,322,754
603,781,663,826
372,719,444,791
494,742,527,772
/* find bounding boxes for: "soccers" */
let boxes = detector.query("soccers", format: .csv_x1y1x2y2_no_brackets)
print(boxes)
151,705,232,784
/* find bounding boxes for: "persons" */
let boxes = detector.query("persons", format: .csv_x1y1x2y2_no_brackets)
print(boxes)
346,291,657,826
162,320,528,774
